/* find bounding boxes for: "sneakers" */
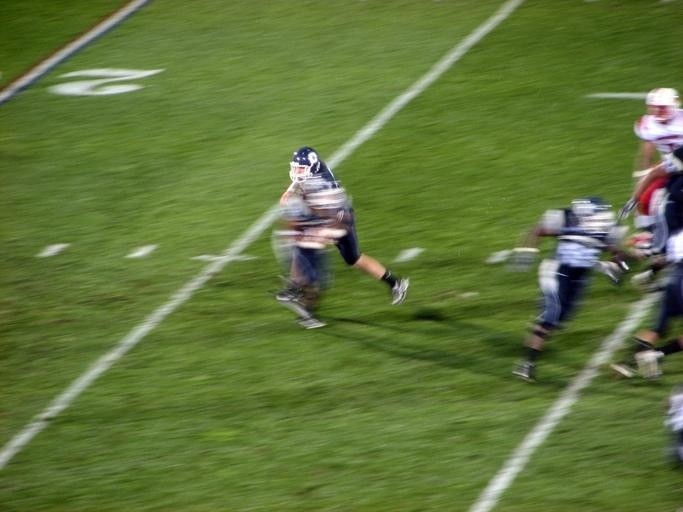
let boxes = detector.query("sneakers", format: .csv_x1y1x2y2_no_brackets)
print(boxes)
513,364,534,379
391,278,408,306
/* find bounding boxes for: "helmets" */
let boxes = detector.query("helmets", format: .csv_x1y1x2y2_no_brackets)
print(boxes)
647,89,678,124
289,147,321,183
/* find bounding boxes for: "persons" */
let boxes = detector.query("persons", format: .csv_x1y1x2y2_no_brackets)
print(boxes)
664,393,683,469
513,88,683,381
274,146,410,330
